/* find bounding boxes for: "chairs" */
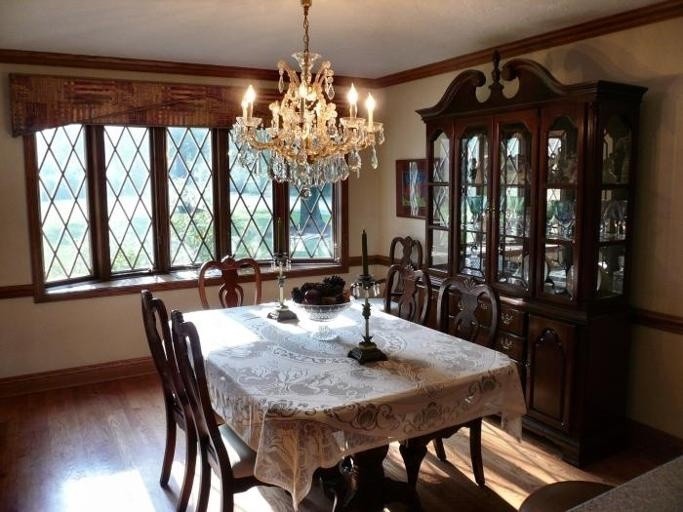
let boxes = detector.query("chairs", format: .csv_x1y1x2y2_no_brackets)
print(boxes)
198,255,262,313
350,236,423,317
382,265,498,490
141,287,346,511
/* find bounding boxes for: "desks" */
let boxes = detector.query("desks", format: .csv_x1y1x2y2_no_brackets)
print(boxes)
168,293,514,511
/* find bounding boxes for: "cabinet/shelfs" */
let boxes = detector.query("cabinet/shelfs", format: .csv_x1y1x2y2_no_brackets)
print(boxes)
417,50,647,469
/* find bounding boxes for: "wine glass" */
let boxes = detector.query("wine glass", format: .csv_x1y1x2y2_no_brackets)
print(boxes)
553,200,574,242
600,200,611,239
612,200,627,241
467,197,482,230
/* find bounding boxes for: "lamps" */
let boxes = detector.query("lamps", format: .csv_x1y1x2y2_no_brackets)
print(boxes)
232,1,385,200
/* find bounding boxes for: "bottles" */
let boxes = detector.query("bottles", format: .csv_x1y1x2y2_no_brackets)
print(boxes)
465,246,481,277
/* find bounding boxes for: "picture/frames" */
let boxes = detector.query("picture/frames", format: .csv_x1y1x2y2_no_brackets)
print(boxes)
395,158,440,220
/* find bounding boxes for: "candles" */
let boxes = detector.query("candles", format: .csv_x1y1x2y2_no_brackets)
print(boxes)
362,227,368,279
